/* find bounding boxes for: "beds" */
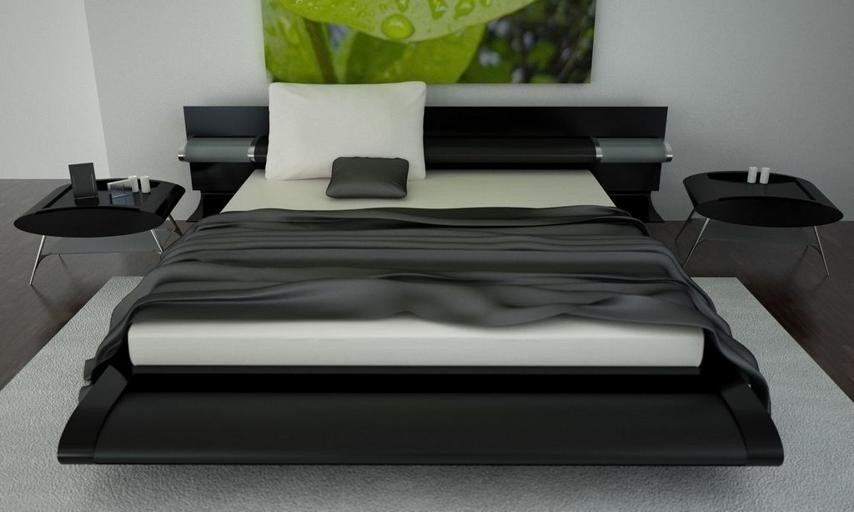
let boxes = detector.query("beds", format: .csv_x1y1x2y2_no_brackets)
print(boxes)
57,106,785,466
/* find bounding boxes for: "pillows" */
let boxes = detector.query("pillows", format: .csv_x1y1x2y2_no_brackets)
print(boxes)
264,81,428,181
325,157,409,199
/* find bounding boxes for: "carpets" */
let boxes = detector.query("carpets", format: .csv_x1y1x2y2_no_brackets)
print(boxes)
0,276,854,512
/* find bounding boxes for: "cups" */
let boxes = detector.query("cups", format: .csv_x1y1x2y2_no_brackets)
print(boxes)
747,167,770,184
129,175,151,193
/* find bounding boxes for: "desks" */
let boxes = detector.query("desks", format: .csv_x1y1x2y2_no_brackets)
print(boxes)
14,178,186,285
674,171,843,279
